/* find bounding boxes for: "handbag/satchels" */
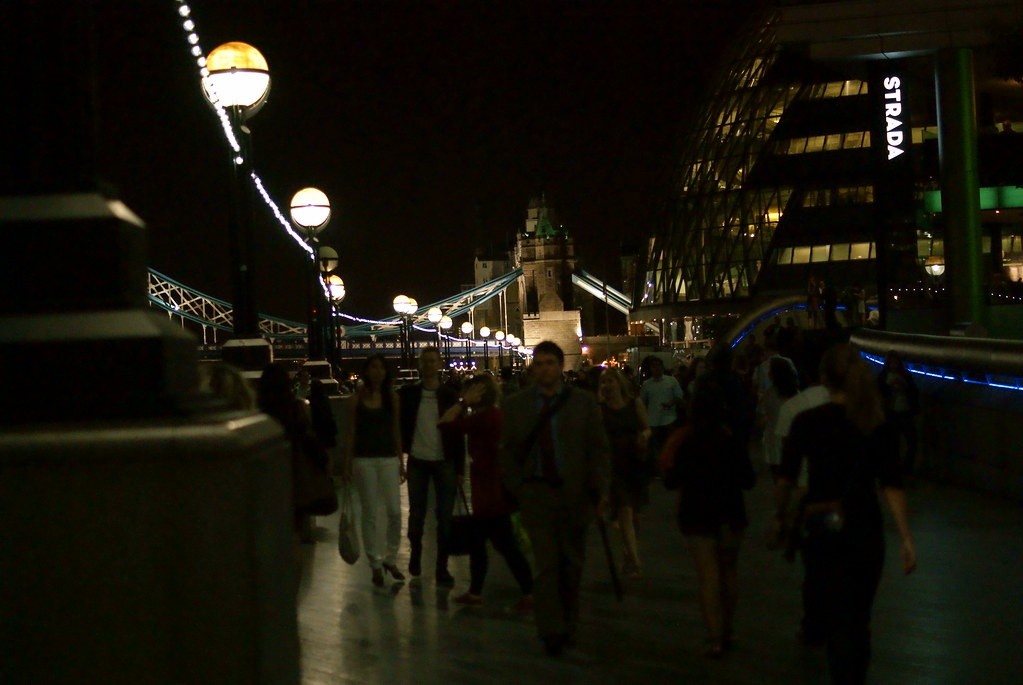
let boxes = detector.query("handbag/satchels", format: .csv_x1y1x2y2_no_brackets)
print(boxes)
338,480,361,563
442,484,474,550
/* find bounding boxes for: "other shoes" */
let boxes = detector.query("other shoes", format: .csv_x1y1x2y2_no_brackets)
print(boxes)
357,537,738,659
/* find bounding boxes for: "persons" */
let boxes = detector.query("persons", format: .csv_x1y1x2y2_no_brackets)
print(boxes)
754,354,804,547
595,366,652,587
397,347,461,588
505,342,613,656
291,370,316,405
337,353,407,589
777,337,845,642
783,341,917,685
436,375,537,614
444,367,470,387
575,357,707,400
666,388,756,655
691,341,756,536
258,363,327,561
878,350,919,481
642,358,689,485
732,264,873,395
497,367,518,395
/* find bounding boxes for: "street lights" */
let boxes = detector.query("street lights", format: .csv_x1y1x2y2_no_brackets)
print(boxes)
428,307,442,352
403,298,420,383
506,333,534,378
318,245,350,396
494,331,505,378
288,185,337,396
462,322,475,380
199,39,274,394
439,316,452,380
480,327,494,376
393,294,414,384
